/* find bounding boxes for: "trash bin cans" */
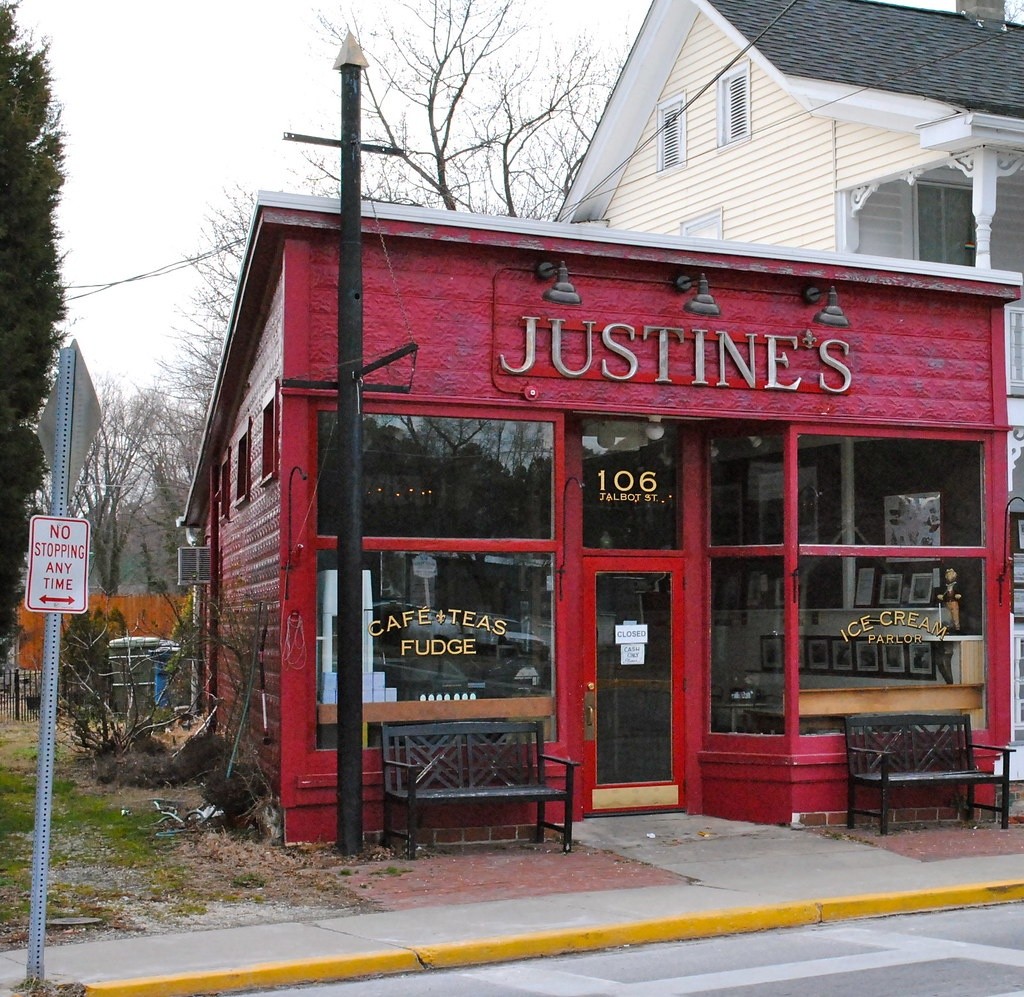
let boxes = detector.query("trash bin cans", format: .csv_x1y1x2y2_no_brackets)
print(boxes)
106,637,180,722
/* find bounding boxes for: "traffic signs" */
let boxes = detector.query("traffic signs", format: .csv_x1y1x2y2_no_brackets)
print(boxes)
24,515,91,614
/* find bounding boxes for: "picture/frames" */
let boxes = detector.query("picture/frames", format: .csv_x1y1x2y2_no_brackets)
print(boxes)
906,571,940,607
782,636,807,674
907,642,936,681
760,634,784,672
773,576,787,609
806,636,831,675
830,637,854,677
853,638,880,678
876,572,905,608
878,638,907,679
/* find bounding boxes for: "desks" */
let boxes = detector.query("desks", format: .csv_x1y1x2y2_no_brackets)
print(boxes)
712,701,771,734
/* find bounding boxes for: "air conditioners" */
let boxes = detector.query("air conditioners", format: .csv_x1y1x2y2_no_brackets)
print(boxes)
177,546,210,586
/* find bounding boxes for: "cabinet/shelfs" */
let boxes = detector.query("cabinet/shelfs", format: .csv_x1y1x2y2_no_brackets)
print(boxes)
710,607,987,731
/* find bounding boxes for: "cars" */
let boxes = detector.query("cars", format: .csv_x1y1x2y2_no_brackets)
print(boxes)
372,600,547,655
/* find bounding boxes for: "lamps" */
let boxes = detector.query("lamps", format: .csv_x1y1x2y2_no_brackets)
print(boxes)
675,273,723,317
803,283,850,328
538,260,582,305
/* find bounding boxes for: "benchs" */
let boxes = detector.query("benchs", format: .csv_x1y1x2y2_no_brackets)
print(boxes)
381,719,579,858
844,712,1015,836
